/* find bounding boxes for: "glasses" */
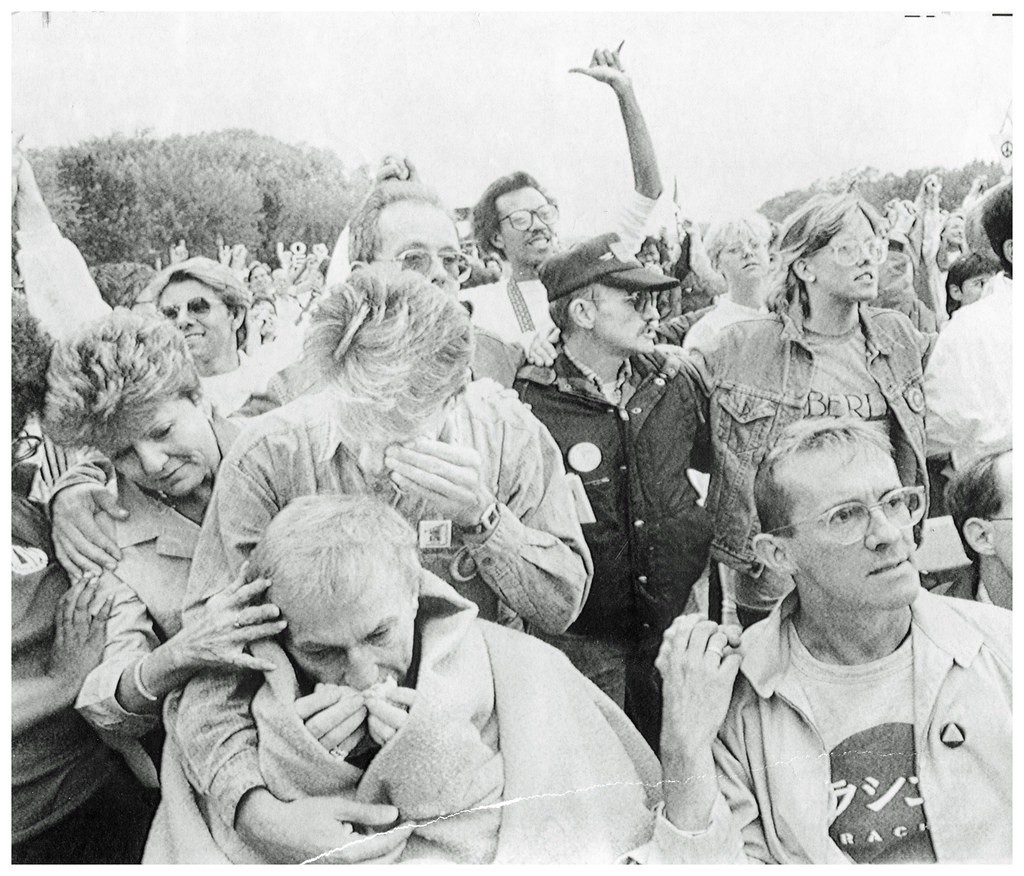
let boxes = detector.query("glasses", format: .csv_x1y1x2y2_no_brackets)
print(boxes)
371,248,473,283
767,484,926,545
11,434,41,462
498,204,560,230
160,296,225,321
586,290,665,313
801,238,890,268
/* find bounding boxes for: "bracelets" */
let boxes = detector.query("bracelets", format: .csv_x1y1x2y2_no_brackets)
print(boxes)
133,650,159,706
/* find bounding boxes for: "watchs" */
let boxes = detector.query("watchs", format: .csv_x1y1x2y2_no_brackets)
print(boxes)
453,498,501,537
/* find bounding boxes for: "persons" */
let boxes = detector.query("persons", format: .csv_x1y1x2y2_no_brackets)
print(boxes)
619,414,1012,864
16,35,1016,864
143,496,656,862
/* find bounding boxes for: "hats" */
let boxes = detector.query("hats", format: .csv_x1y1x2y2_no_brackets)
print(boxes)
535,232,680,294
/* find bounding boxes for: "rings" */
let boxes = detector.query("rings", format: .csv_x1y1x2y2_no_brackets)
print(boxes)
708,646,725,658
